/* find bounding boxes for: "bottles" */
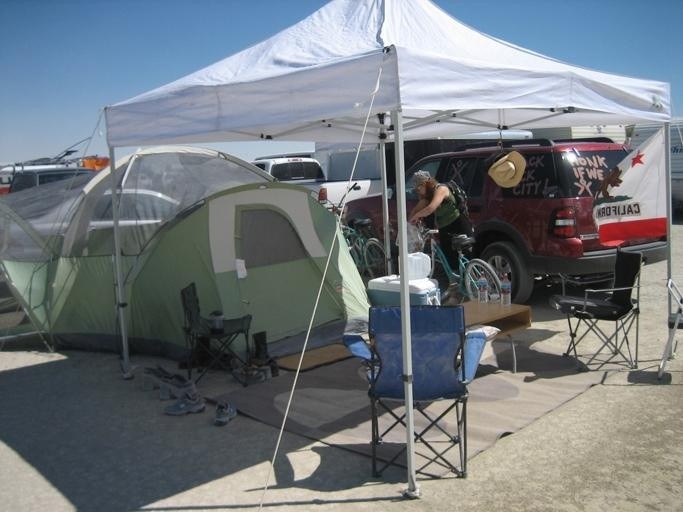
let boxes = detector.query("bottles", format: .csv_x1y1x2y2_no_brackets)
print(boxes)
478,271,511,307
209,311,224,334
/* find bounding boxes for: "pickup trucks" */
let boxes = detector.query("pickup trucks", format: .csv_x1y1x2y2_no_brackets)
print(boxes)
250,157,382,212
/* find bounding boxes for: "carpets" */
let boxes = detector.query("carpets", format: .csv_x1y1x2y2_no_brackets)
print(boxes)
205,339,629,477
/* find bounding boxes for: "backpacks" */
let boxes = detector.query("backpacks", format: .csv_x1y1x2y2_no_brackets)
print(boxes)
436,180,470,218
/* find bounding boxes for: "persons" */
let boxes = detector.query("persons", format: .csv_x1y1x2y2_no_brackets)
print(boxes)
408,169,481,306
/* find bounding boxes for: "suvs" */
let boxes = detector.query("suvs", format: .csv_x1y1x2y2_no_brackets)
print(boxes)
341,137,668,305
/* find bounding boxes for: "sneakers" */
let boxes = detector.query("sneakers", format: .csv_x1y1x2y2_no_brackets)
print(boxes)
214,399,237,425
165,395,206,415
232,358,279,384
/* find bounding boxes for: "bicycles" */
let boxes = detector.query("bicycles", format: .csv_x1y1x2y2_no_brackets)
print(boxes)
327,182,396,279
417,218,501,303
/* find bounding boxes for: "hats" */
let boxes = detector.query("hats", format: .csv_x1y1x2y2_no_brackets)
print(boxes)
488,151,526,188
413,170,431,188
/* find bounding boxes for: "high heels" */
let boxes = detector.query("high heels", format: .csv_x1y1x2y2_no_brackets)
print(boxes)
142,367,195,400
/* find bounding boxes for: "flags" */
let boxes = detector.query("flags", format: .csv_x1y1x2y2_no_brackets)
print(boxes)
591,126,668,248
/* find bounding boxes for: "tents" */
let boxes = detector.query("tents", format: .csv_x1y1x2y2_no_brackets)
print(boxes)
1,143,372,371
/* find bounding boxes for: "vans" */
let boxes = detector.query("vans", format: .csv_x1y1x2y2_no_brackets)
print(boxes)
10,168,95,193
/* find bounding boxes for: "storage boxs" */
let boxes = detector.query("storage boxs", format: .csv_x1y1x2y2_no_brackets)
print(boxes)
365,274,439,307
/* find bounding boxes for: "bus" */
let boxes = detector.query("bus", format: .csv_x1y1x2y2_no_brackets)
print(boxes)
0,163,77,195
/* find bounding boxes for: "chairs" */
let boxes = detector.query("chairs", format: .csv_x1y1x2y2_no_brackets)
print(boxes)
548,249,642,370
340,305,486,479
